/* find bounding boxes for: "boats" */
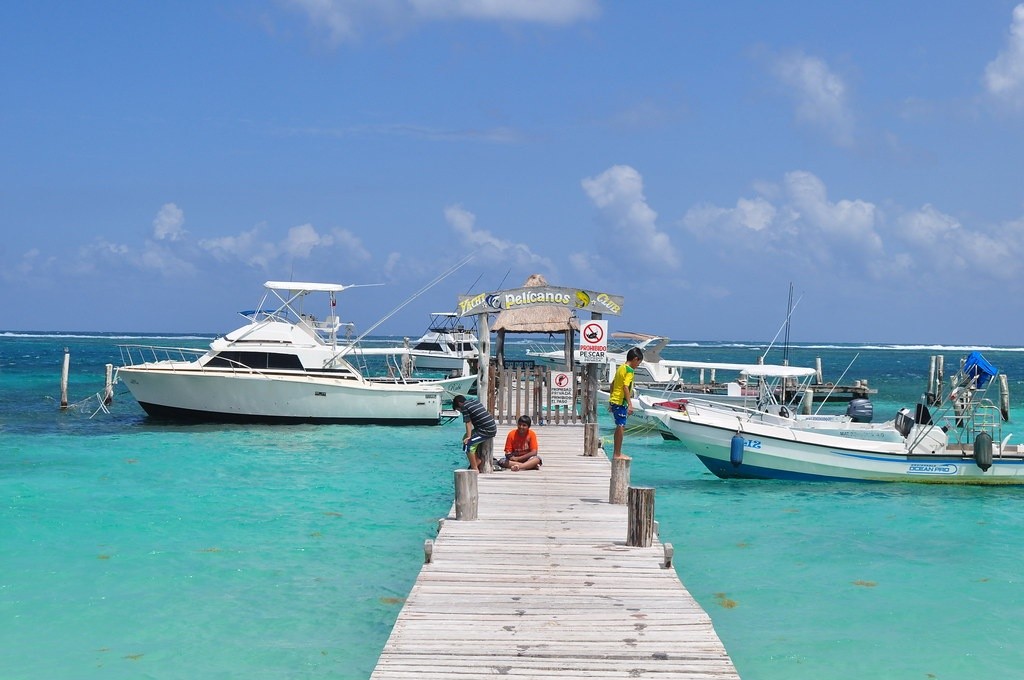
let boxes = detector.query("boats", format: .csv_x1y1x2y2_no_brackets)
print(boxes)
524,282,945,442
643,346,1024,488
409,264,507,372
112,279,478,425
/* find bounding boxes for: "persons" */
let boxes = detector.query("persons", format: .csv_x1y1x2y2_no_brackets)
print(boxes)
498,415,542,472
607,347,643,460
452,395,497,474
